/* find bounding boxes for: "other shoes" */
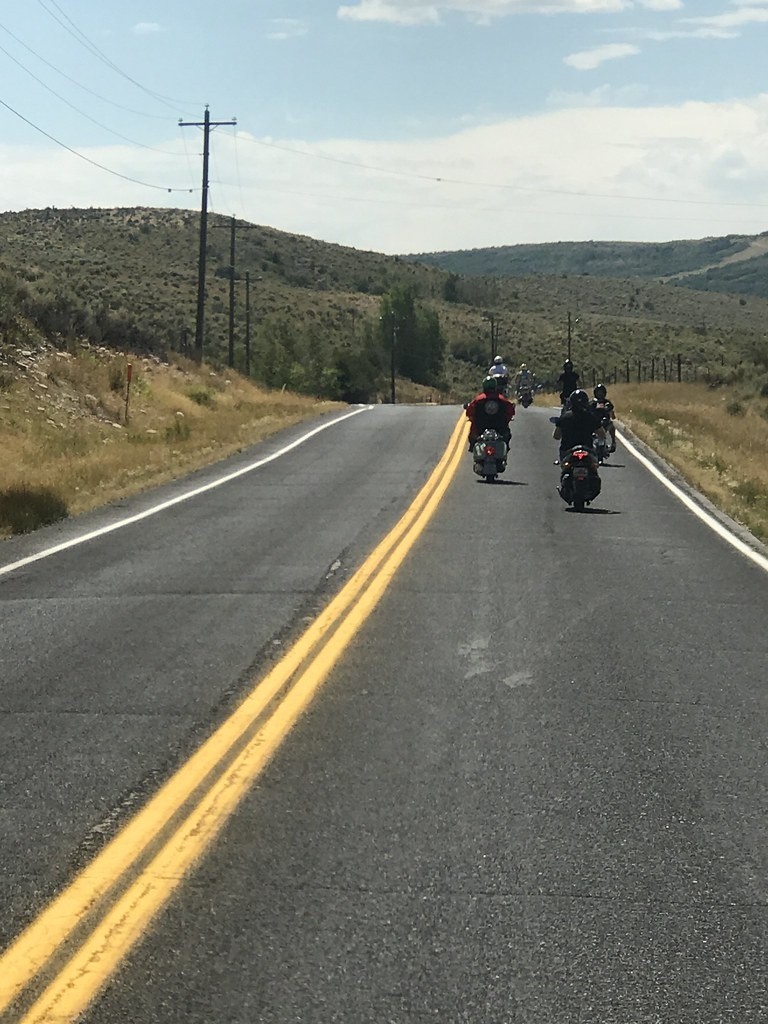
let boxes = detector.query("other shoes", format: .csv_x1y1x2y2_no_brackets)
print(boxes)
611,442,617,452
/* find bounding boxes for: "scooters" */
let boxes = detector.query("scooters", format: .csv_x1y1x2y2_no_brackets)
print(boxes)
463,400,515,482
487,371,615,463
549,413,605,510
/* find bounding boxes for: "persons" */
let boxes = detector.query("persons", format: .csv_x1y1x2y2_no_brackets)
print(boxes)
559,359,580,405
514,363,533,395
588,384,617,454
488,355,509,375
553,389,605,459
466,375,515,453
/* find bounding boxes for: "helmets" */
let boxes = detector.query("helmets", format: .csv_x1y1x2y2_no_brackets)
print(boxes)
564,358,573,371
520,364,528,371
570,390,588,407
494,356,503,364
594,384,606,398
482,376,497,389
